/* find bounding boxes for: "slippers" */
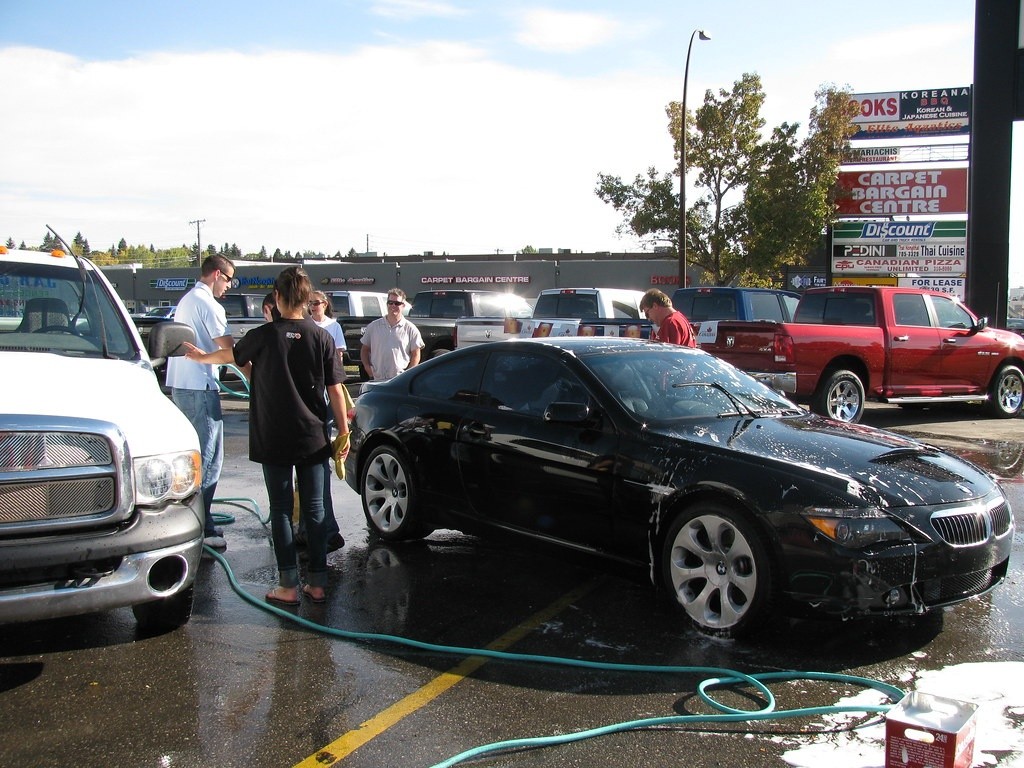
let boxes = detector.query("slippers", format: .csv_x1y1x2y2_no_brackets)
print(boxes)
265,587,300,606
301,584,325,603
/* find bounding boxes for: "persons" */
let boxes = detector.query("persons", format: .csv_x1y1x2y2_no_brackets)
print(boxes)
640,290,696,349
360,288,425,382
165,255,253,547
184,266,350,604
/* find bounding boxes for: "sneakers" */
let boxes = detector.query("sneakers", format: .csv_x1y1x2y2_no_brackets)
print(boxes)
204,526,227,547
293,533,345,562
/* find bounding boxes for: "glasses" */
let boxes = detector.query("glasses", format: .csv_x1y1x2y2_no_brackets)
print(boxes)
387,301,404,306
645,308,650,316
307,300,324,306
220,270,232,282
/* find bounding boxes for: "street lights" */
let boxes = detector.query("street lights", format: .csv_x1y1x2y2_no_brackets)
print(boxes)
678,29,711,287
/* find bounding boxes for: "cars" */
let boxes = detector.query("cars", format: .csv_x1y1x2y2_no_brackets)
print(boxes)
342,335,1016,638
142,307,177,318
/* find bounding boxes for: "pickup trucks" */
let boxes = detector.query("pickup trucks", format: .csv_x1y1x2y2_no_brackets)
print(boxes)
228,291,412,342
453,288,649,349
132,294,270,381
504,288,801,339
688,285,1023,424
0,247,204,632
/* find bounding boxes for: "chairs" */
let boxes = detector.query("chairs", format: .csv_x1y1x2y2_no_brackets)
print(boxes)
15,299,71,334
608,363,648,413
